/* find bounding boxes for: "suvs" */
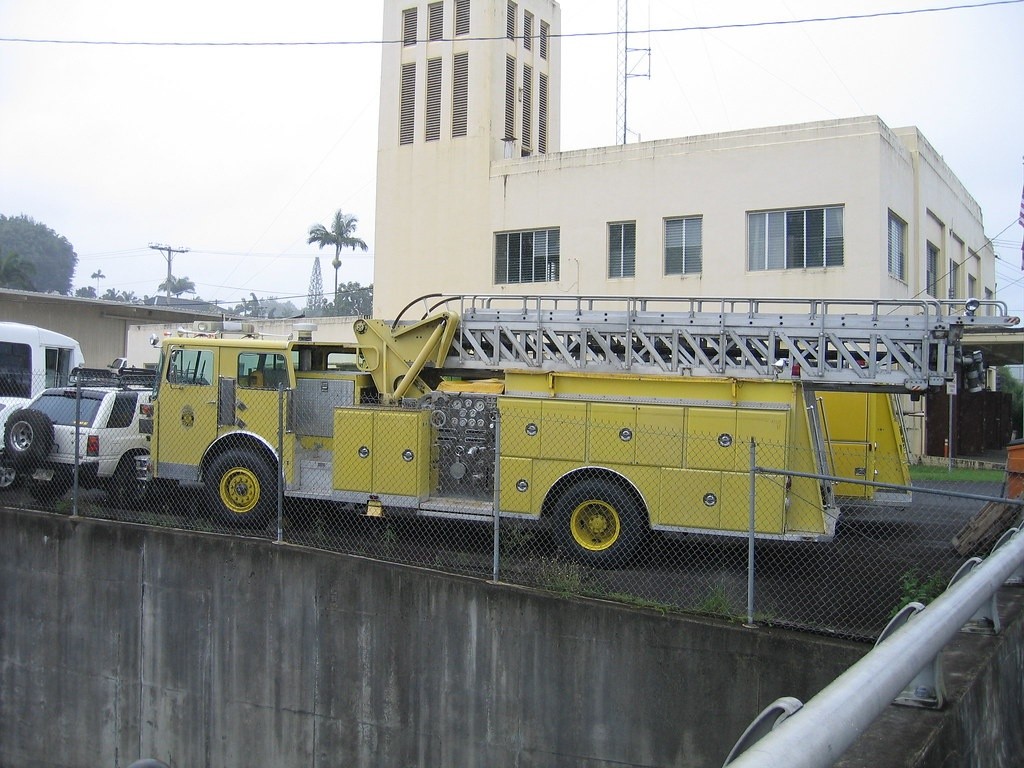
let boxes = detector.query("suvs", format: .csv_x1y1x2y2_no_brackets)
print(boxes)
5,356,178,509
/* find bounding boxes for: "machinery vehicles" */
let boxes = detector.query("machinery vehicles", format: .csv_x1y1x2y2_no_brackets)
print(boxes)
149,290,1020,571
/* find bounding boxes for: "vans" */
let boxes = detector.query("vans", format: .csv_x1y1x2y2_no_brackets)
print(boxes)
0,321,85,490
